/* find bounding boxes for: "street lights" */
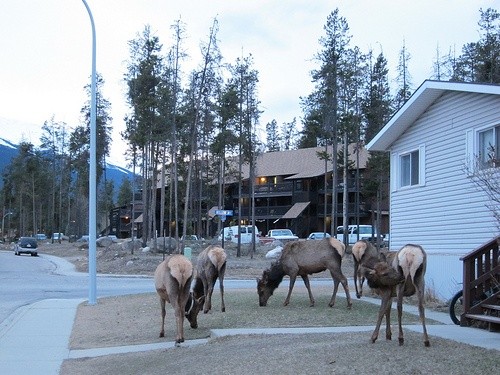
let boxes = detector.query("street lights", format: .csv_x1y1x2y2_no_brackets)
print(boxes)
2,212,13,243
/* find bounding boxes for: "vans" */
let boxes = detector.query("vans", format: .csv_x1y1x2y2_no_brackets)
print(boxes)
334,224,384,248
52,232,63,242
218,224,260,243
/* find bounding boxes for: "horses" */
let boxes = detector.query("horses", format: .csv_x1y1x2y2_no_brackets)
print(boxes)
351,239,431,348
255,238,352,309
154,244,227,343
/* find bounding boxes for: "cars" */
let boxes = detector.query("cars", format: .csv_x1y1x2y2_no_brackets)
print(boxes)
15,237,38,256
307,232,332,241
35,234,47,240
265,229,300,244
